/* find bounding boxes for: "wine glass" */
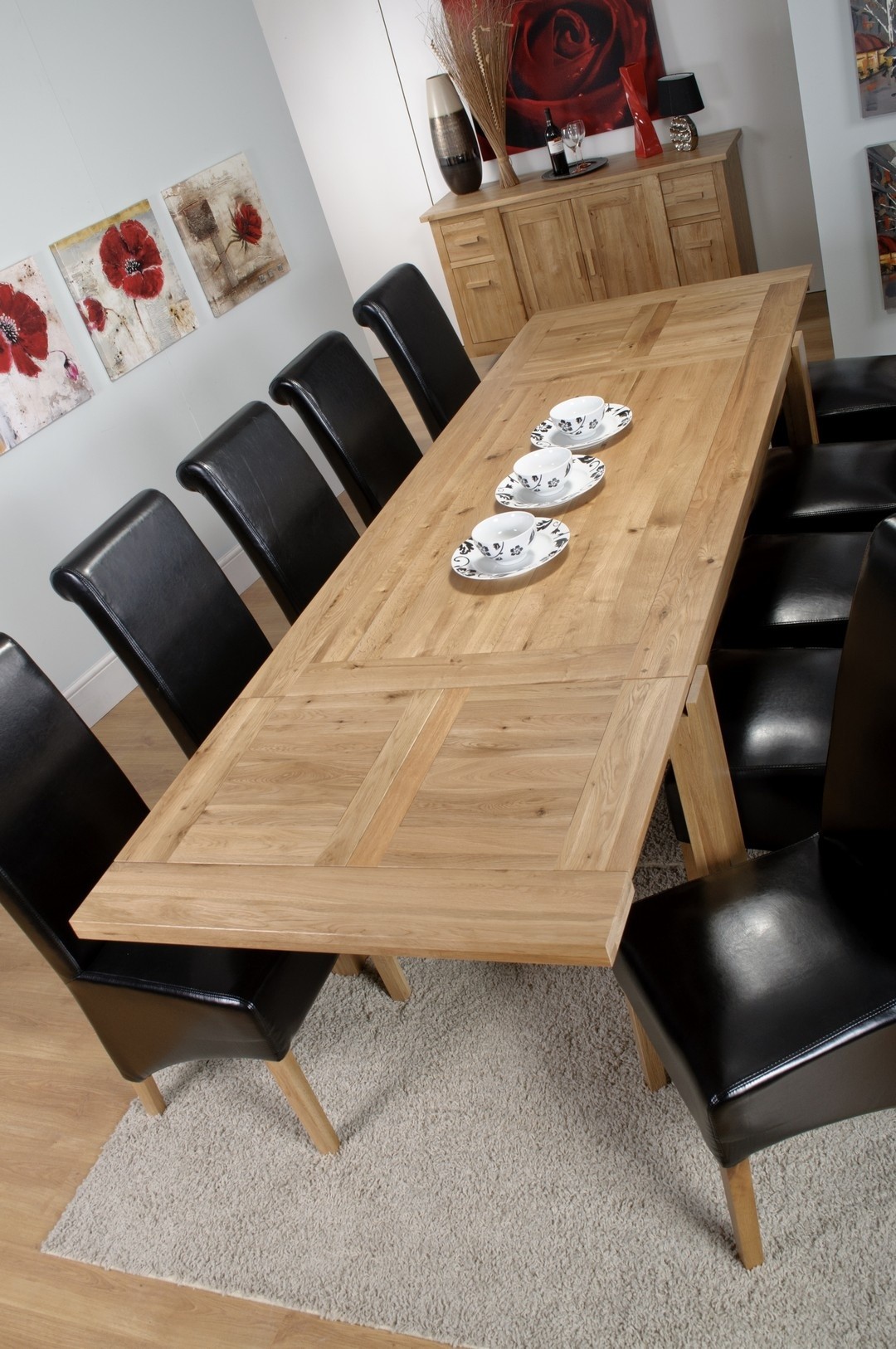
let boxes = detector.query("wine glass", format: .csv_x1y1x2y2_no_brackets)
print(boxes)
561,126,587,174
567,120,591,168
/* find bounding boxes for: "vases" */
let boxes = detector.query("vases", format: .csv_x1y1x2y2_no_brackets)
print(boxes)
426,73,483,196
619,62,663,159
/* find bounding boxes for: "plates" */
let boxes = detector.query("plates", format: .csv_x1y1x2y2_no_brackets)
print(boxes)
531,403,633,450
451,517,570,581
541,157,608,179
494,454,604,508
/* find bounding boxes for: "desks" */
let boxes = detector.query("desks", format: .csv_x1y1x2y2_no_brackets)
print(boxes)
69,264,819,968
417,128,742,219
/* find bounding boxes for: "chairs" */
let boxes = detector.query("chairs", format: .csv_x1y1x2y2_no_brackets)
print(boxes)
0,262,884,1271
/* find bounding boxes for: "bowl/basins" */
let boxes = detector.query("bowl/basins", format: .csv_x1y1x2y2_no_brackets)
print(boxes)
513,447,573,496
471,510,537,562
548,395,605,440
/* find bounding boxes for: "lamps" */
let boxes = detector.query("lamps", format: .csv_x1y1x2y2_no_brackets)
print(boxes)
657,72,705,152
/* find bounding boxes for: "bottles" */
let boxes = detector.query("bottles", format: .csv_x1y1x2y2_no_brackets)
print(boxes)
543,107,569,176
619,62,663,159
426,73,483,196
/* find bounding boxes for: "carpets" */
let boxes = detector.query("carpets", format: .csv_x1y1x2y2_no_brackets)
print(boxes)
40,769,896,1349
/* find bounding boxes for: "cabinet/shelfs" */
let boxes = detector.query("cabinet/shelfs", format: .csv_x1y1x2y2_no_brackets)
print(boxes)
429,147,759,359
